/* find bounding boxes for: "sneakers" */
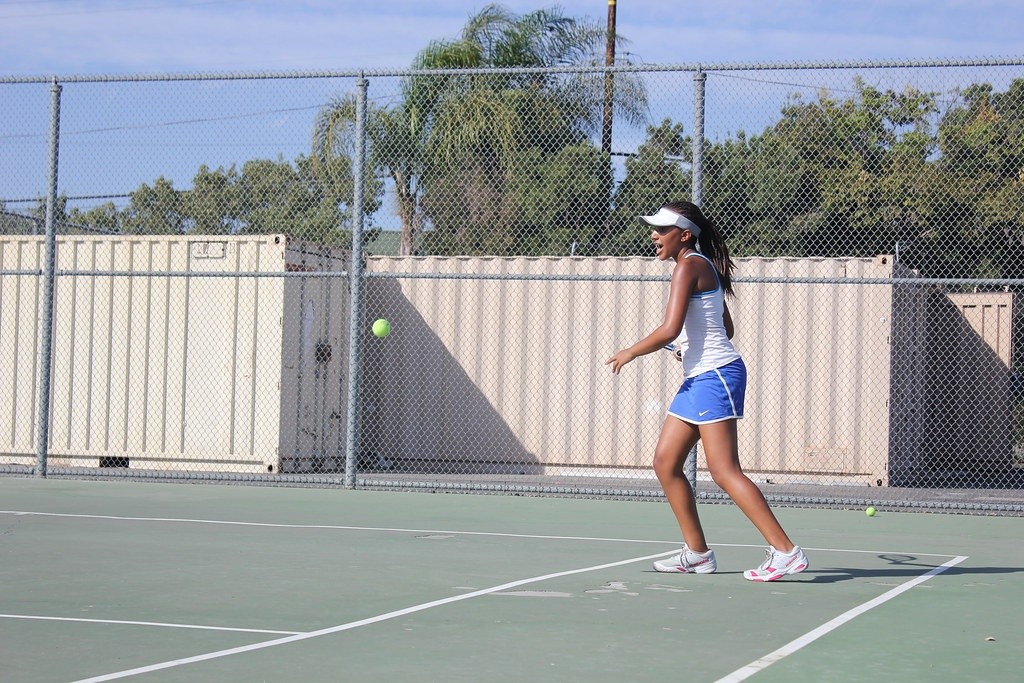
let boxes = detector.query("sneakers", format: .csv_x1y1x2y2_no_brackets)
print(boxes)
653,543,717,574
743,545,808,582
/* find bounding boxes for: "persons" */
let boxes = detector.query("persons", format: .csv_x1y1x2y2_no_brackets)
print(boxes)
605,201,811,583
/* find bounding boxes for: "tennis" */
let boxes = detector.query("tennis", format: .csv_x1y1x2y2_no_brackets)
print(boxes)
373,319,390,337
865,507,875,517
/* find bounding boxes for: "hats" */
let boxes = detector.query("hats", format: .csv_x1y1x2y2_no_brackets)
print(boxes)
639,208,702,238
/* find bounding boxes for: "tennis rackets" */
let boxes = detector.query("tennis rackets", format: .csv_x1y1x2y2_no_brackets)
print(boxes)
664,343,683,361
